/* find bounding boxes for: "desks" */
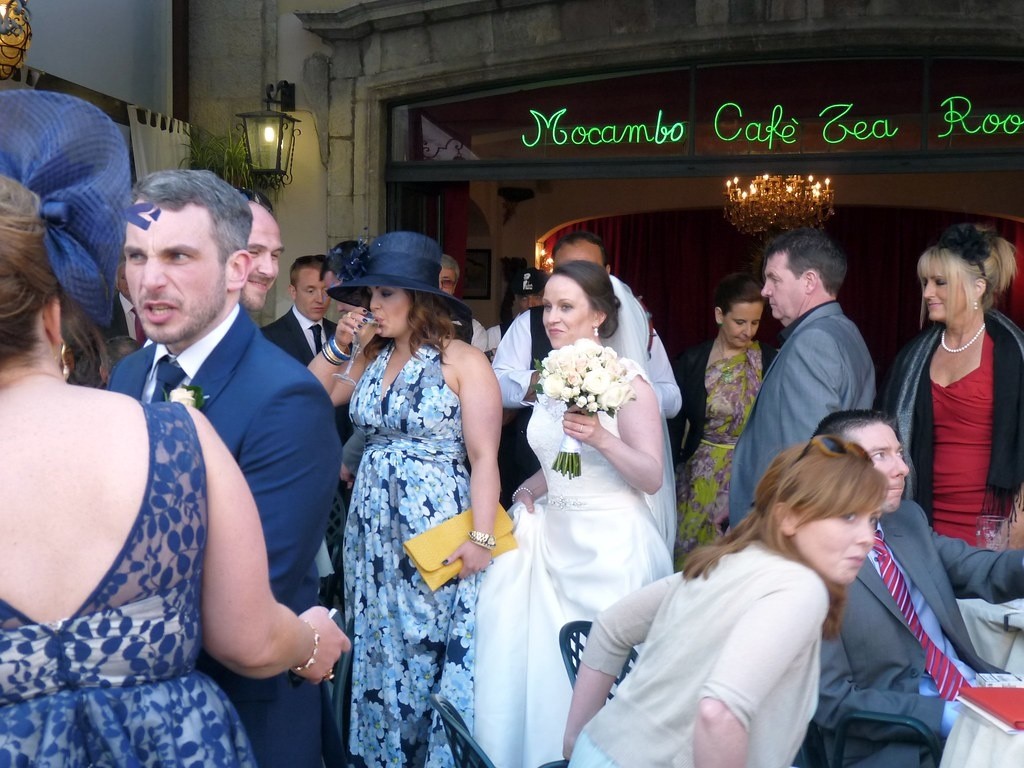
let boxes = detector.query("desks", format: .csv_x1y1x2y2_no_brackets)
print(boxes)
940,704,1024,768
955,595,1024,673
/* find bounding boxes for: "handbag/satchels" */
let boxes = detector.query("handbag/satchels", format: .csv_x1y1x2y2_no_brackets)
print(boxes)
404,503,519,593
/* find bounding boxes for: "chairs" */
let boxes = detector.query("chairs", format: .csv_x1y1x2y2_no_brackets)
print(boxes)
557,618,643,706
426,688,571,768
798,706,940,768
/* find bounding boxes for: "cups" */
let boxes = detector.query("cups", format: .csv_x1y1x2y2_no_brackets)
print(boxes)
976,515,1011,552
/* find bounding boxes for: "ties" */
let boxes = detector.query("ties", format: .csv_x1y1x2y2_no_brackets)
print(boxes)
149,359,186,404
872,531,972,702
308,323,322,355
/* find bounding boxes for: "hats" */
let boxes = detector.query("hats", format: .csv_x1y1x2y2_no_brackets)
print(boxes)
324,231,472,320
512,268,544,294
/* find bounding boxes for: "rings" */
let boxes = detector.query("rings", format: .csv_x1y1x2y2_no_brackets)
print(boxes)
347,312,353,318
579,424,584,432
323,669,334,681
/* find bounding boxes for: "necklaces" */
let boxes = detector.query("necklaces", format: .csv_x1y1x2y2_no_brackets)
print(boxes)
716,339,734,370
941,323,986,353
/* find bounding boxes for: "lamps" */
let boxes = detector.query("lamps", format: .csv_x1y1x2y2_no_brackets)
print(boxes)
0,0,35,83
234,79,301,193
720,117,838,242
539,247,555,272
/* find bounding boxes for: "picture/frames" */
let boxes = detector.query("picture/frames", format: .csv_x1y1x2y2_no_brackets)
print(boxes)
463,248,492,300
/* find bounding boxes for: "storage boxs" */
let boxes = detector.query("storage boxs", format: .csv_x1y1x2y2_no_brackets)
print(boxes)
975,673,1024,688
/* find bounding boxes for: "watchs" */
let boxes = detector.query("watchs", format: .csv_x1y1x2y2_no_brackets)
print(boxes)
468,530,496,550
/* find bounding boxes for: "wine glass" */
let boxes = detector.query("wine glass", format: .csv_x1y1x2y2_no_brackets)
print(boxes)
332,311,382,386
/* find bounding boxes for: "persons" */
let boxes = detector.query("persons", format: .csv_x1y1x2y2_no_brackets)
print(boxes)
1,88,1024,768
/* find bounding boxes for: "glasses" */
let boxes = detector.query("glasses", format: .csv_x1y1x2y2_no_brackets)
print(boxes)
440,279,457,291
794,435,874,474
236,187,274,213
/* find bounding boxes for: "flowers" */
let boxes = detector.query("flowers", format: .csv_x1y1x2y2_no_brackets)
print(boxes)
528,333,626,480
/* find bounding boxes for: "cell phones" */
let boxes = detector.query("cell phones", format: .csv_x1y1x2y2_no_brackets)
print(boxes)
284,608,345,689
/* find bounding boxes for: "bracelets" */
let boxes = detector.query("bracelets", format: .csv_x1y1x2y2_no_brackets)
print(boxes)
321,336,352,366
512,486,533,502
291,620,321,672
